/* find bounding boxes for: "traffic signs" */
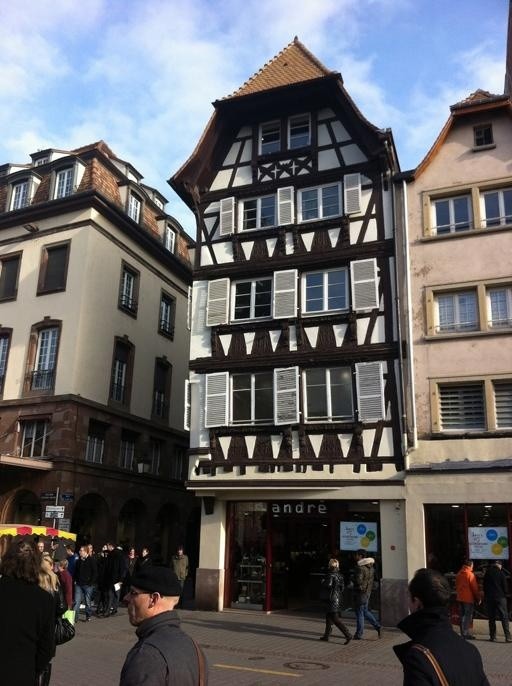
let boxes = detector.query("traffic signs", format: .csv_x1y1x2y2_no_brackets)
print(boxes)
43,506,65,519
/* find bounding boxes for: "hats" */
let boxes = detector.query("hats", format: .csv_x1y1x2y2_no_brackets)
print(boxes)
129,564,183,597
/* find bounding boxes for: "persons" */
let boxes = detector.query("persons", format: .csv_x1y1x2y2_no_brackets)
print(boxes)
0,547,77,684
2,539,193,627
115,547,211,686
318,555,352,640
392,565,494,685
349,547,384,640
480,556,511,646
455,560,480,642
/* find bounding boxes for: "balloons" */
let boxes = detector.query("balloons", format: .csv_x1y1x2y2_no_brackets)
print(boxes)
365,530,375,542
356,524,367,535
486,529,497,542
496,535,508,548
491,543,502,555
360,537,369,548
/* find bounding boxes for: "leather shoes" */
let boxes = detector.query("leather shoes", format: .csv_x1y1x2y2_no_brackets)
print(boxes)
377,625,384,639
319,635,327,640
344,634,353,644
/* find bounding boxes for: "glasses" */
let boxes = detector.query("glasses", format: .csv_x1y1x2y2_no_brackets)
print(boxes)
130,591,146,597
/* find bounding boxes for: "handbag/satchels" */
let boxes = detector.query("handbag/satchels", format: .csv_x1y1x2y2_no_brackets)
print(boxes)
321,575,337,604
